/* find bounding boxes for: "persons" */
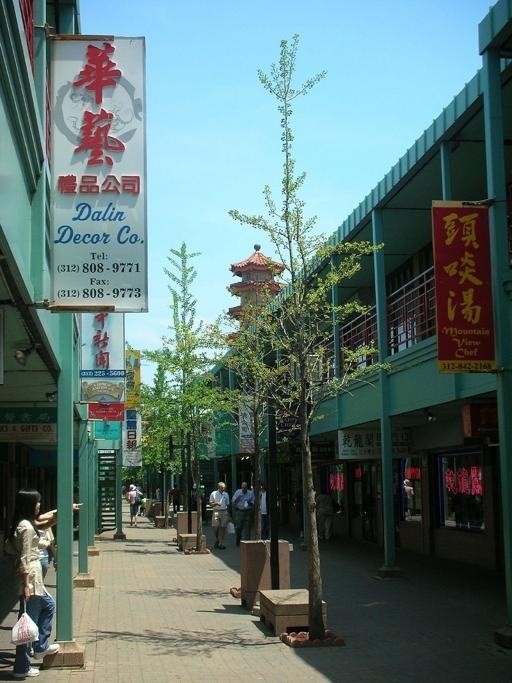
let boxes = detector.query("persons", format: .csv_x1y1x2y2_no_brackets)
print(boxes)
403,478,417,518
258,483,272,541
155,487,161,500
34,508,58,582
8,488,86,679
208,481,231,550
230,480,257,547
314,488,336,539
126,483,144,528
172,482,180,513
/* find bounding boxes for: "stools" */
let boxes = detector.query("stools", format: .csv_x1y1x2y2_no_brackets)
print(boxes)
155,516,172,527
178,534,206,551
260,588,327,638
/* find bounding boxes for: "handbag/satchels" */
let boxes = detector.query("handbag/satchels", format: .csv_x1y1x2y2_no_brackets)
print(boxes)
226,520,236,535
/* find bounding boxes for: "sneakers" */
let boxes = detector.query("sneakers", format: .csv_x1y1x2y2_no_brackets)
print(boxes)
213,541,226,550
33,643,60,660
11,666,40,679
